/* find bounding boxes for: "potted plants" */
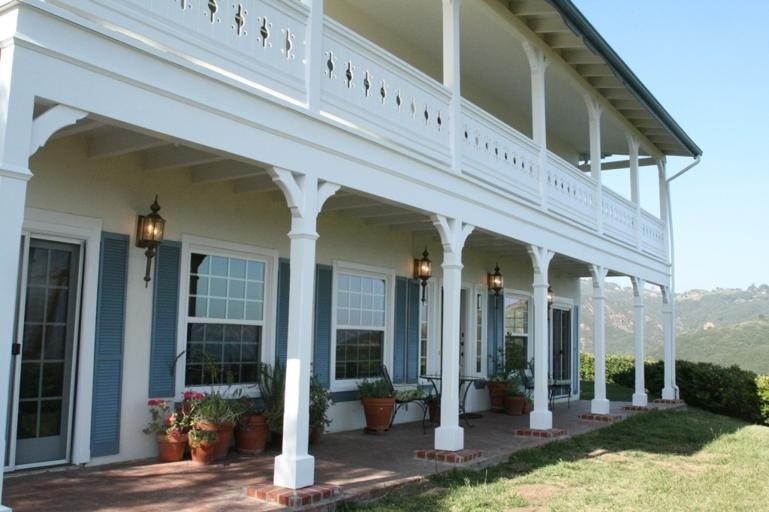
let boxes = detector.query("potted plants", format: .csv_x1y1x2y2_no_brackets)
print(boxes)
427,394,440,423
189,347,336,465
488,330,534,415
356,377,395,435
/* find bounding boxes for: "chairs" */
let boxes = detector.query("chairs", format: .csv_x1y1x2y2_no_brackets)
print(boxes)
548,350,571,411
380,364,433,435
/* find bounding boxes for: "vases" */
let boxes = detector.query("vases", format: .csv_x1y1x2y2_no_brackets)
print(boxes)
156,432,187,461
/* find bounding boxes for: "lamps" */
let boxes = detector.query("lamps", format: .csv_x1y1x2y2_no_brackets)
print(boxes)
135,193,167,289
547,285,554,320
487,261,503,309
413,242,433,305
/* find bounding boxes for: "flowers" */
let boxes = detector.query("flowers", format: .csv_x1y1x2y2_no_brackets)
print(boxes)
141,388,202,442
